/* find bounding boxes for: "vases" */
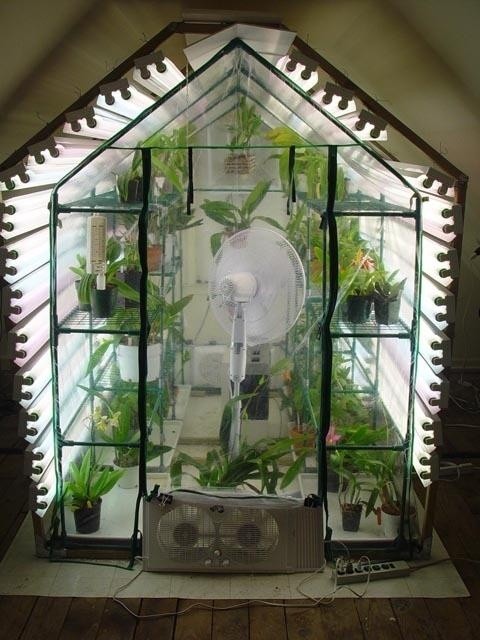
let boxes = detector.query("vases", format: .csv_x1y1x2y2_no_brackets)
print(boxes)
372,295,402,326
346,293,371,325
282,385,295,399
111,459,140,489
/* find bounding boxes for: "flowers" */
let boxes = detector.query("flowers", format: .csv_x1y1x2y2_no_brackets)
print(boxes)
335,247,406,297
281,370,295,385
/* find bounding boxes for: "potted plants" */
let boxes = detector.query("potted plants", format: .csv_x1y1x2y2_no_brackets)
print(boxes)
57,448,129,537
354,458,414,538
120,226,142,309
222,95,264,175
199,178,289,266
71,234,138,318
108,122,193,206
76,376,173,467
335,463,380,534
278,395,315,457
144,205,203,270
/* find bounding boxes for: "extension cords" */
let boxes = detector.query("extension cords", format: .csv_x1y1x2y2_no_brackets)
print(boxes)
332,560,411,584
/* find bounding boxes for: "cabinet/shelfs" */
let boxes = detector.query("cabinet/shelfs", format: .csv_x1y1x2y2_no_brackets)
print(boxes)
47,145,194,538
287,144,429,541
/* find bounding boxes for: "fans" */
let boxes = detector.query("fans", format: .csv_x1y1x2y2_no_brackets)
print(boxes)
206,226,308,465
